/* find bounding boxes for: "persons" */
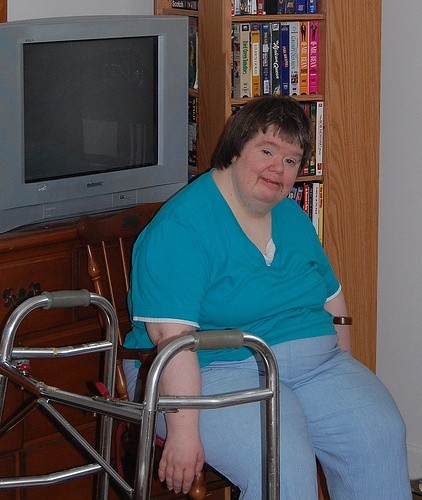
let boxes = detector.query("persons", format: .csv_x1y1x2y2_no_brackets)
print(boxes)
121,93,413,500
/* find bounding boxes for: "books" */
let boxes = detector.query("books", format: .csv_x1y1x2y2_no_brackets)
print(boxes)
172,0,324,246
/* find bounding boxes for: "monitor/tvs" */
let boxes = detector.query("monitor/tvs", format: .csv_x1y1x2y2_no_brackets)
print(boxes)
0,15,189,234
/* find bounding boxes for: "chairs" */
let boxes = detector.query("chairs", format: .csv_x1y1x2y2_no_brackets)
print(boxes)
79,201,354,500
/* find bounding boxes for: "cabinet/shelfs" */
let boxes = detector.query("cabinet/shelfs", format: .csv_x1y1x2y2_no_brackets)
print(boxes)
0,199,234,500
153,0,326,248
